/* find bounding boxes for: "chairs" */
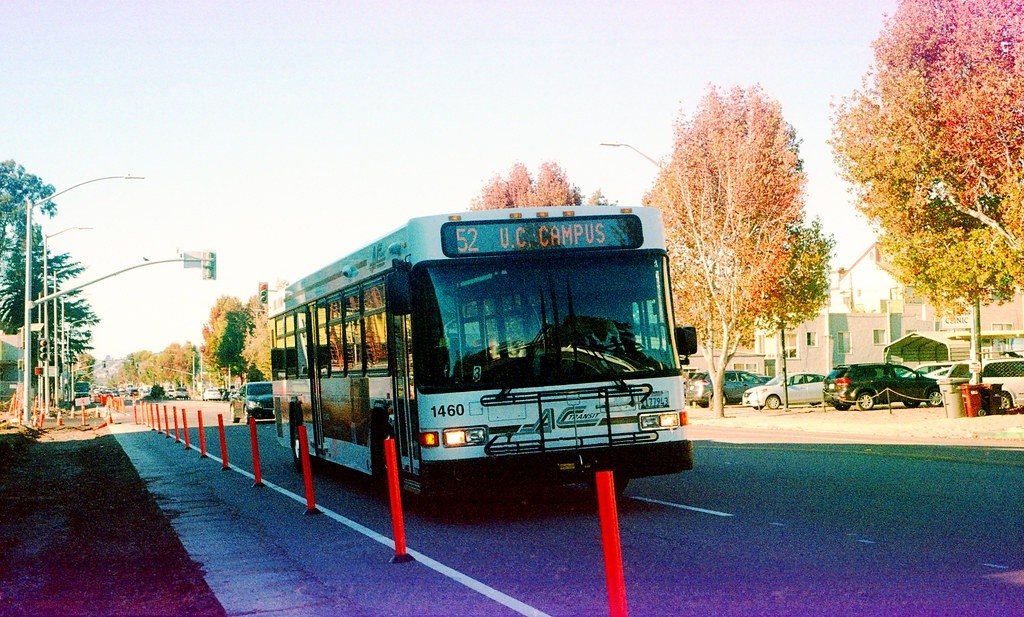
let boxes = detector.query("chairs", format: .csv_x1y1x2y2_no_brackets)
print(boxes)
798,377,803,384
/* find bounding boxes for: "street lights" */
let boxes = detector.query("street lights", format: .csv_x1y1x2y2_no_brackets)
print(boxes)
42,226,93,417
23,175,144,427
53,264,81,411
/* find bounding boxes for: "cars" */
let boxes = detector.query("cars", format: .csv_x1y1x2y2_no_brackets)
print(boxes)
202,387,239,402
903,358,1024,416
741,372,827,411
682,365,779,386
229,381,276,425
91,383,190,406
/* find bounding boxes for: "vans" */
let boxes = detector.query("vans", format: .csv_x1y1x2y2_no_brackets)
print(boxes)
0,359,38,410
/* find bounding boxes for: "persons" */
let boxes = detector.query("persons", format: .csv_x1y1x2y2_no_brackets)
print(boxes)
586,313,626,351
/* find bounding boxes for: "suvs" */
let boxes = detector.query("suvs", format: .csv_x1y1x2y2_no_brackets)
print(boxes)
822,362,945,412
685,368,766,408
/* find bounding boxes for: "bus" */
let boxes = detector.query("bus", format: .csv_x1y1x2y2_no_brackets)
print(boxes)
268,204,696,509
69,381,92,399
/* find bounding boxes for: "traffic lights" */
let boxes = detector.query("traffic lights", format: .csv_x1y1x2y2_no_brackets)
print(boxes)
258,281,268,305
37,338,48,361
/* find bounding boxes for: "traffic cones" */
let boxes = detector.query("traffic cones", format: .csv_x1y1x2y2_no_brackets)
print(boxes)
32,416,40,427
56,411,63,427
94,405,101,418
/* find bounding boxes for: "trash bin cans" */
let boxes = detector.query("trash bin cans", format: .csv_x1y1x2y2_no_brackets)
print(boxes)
960,384,986,417
936,378,970,418
981,383,1003,416
